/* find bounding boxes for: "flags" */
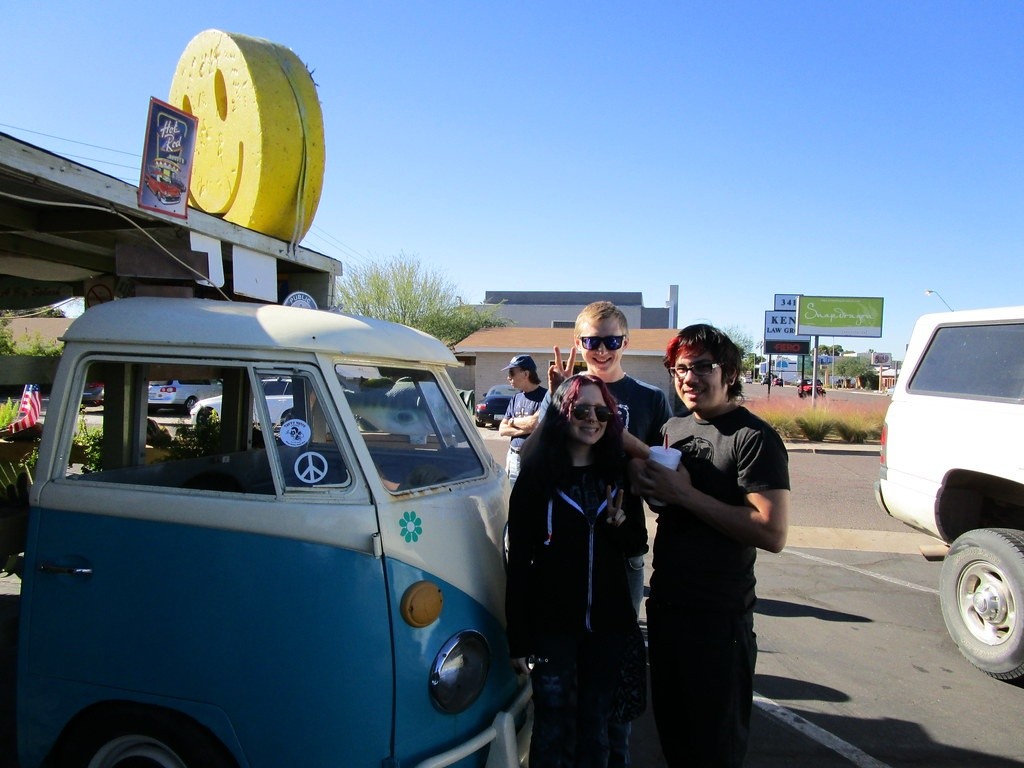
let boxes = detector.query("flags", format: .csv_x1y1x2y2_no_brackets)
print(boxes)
8,384,41,434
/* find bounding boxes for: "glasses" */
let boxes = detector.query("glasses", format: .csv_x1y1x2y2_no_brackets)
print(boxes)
581,335,625,351
670,363,724,377
571,406,613,422
509,371,521,377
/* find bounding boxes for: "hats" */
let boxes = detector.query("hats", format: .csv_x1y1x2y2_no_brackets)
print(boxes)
501,355,535,371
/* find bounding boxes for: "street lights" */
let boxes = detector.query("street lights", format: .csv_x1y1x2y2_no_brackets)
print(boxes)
925,288,955,312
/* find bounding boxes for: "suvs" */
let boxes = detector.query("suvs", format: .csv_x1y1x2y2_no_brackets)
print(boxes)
872,305,1024,683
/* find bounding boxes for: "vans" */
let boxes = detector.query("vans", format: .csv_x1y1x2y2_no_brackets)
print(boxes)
1,293,537,768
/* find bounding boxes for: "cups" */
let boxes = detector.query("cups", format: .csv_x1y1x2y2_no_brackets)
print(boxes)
648,446,682,506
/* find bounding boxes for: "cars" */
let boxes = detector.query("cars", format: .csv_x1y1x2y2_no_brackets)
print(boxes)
188,376,296,426
81,382,105,408
761,378,769,385
146,379,223,416
796,379,827,399
771,378,783,387
475,385,522,428
742,377,752,384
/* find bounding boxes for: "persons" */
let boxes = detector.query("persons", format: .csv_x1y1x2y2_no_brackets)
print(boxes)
499,356,548,489
506,375,649,768
539,301,672,611
640,325,791,768
